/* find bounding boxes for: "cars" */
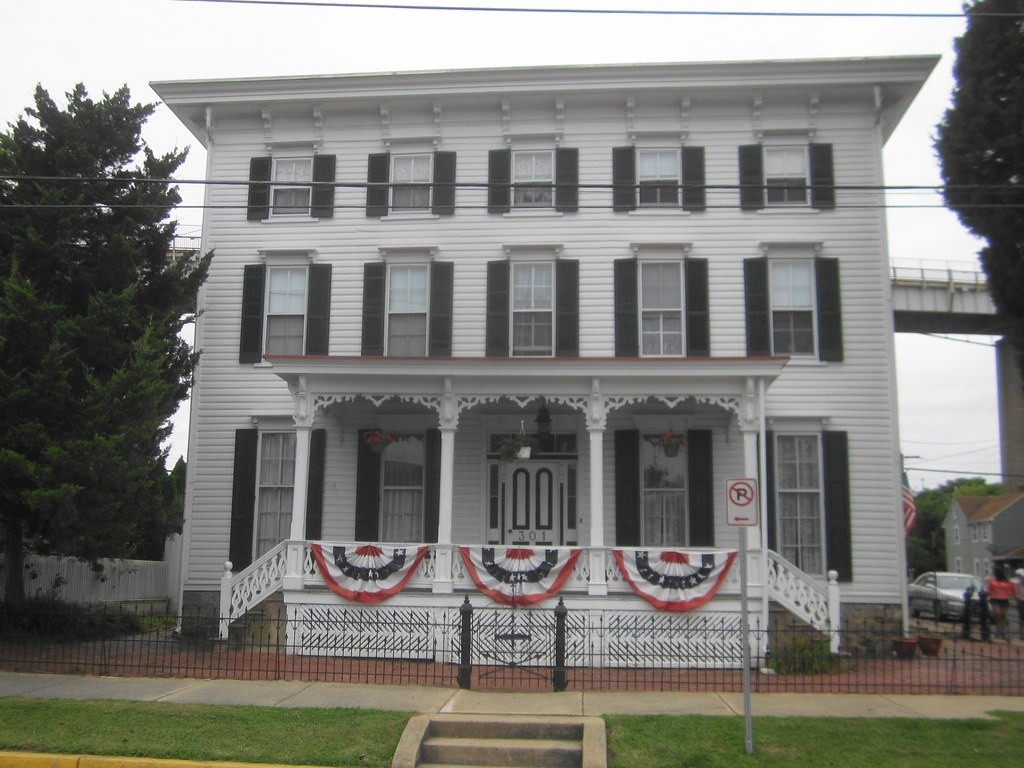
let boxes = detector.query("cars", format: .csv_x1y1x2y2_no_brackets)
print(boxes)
908,572,985,622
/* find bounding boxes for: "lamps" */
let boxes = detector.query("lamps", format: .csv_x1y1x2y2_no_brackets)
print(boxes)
533,408,553,440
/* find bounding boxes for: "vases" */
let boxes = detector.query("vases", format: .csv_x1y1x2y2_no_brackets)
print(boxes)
369,443,384,454
918,633,944,656
664,445,680,457
891,636,919,658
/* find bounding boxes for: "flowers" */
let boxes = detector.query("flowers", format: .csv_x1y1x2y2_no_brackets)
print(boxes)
659,432,688,447
363,431,391,444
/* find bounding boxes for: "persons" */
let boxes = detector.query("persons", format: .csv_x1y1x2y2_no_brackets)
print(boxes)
1012,568,1024,640
985,566,1024,638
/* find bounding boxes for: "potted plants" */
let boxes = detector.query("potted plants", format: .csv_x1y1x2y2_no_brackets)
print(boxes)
498,436,541,463
644,464,669,489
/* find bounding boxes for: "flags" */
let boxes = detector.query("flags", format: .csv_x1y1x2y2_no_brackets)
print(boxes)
902,472,916,537
